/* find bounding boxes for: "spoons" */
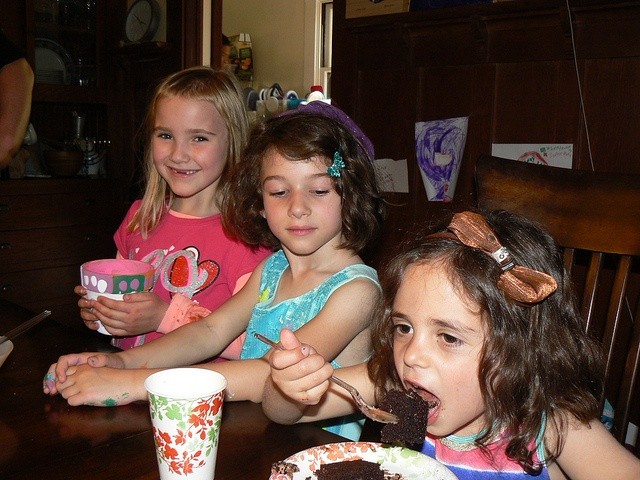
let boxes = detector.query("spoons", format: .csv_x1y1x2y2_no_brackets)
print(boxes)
254,332,400,425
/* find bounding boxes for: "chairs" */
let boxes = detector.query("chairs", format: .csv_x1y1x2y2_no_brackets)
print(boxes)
474,156,640,460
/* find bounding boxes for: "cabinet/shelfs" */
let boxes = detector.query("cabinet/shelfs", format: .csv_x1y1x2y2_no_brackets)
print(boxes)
0,178,113,309
26,1,106,102
331,1,639,458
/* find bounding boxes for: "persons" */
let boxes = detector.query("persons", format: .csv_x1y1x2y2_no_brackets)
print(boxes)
0,38,34,167
262,204,640,479
220,32,232,68
43,102,392,441
73,67,275,364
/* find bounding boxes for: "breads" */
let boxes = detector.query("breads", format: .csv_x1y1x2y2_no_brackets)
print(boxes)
380,388,429,453
313,459,384,480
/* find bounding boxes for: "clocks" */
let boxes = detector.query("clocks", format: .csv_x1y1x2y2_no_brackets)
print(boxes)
124,1,161,42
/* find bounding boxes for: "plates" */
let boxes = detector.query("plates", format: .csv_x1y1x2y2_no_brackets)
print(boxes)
267,440,460,480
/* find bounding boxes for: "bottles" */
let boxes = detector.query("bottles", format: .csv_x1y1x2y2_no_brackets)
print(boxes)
81,141,101,178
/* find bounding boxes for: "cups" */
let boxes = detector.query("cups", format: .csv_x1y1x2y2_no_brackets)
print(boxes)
143,367,227,480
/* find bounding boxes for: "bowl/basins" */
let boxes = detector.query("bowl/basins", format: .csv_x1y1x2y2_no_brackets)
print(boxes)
80,258,156,336
